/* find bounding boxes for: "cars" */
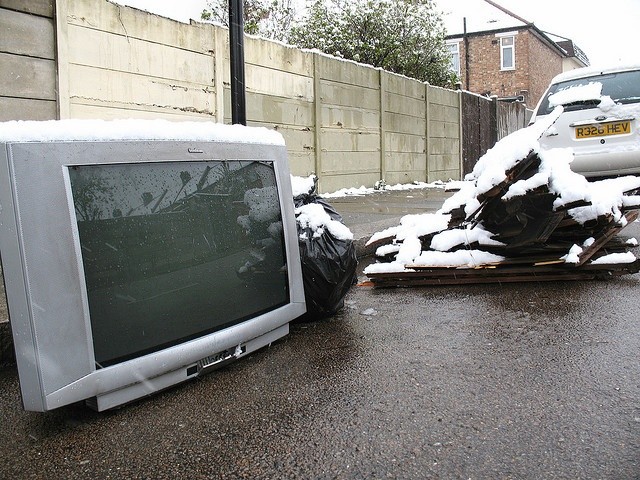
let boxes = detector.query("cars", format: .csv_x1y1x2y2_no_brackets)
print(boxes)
526,64,640,178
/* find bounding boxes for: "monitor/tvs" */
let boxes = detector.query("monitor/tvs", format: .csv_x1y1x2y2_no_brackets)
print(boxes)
1,140,307,412
